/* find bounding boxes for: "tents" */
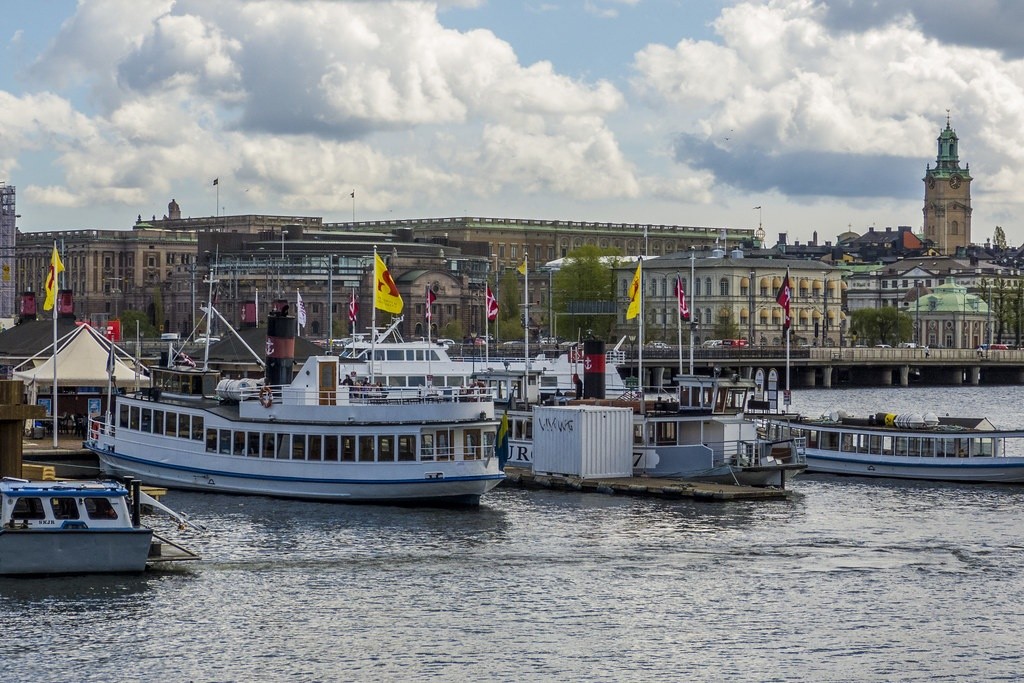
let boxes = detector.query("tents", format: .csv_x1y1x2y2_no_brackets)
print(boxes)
12,328,152,434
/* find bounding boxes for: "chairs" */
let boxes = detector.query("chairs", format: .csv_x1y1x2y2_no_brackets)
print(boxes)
47,413,87,440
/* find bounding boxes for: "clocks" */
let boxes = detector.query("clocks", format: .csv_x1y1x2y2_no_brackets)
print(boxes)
928,175,961,190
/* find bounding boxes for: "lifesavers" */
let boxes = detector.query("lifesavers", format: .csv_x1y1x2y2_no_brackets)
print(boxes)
259,386,273,408
92,422,100,440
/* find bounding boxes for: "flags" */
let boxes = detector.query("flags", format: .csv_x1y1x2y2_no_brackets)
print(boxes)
777,270,791,330
351,192,355,198
349,293,358,322
425,287,437,322
297,292,306,328
674,277,690,319
516,257,527,276
213,178,219,185
485,286,500,322
496,412,509,472
373,252,404,315
626,260,642,320
106,344,116,374
43,246,66,311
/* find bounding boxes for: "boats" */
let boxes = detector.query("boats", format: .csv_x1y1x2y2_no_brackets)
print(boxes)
0,474,207,574
84,249,1024,509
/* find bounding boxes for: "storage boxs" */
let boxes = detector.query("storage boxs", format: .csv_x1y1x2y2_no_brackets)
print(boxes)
530,403,634,480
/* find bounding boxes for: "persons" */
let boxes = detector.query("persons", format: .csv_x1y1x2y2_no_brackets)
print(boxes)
924,345,929,359
554,343,559,358
959,448,965,457
74,413,88,439
92,498,116,519
554,389,563,396
750,395,754,401
342,372,384,397
977,346,984,358
459,384,468,395
